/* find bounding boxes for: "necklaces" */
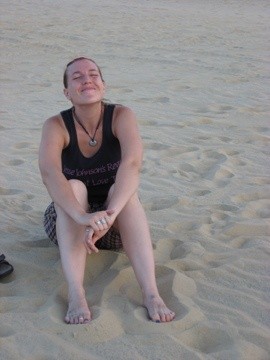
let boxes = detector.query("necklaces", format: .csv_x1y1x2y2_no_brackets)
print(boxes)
73,103,104,146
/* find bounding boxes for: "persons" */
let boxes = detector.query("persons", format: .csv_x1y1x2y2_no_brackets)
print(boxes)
38,57,176,325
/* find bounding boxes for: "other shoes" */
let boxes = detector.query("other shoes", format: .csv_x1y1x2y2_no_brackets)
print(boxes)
0,254,13,278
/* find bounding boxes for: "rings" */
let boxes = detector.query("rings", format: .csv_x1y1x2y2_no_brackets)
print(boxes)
96,221,101,225
101,218,106,224
85,228,90,232
